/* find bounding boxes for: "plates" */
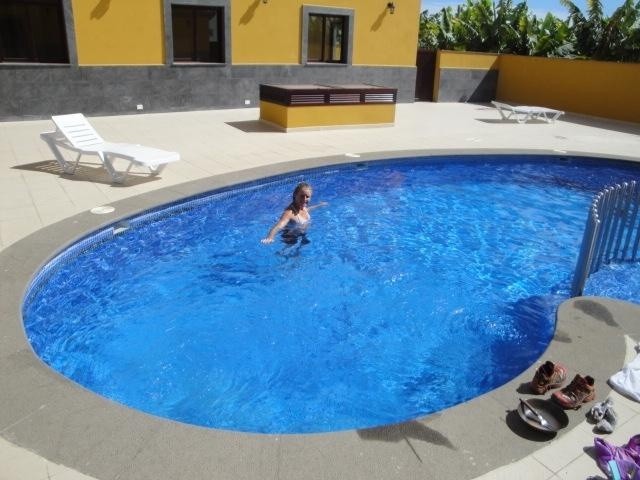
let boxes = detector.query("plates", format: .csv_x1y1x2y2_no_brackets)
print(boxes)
516,397,570,433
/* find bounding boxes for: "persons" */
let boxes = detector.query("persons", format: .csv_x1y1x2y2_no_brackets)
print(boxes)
259,182,329,245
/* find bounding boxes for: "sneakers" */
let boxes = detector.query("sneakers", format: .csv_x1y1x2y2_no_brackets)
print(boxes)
551,373,595,411
530,360,568,395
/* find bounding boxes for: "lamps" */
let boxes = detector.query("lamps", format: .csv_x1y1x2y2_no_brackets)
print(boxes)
387,2,395,14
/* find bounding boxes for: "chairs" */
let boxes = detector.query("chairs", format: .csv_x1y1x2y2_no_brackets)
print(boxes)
491,101,565,124
39,113,179,184
